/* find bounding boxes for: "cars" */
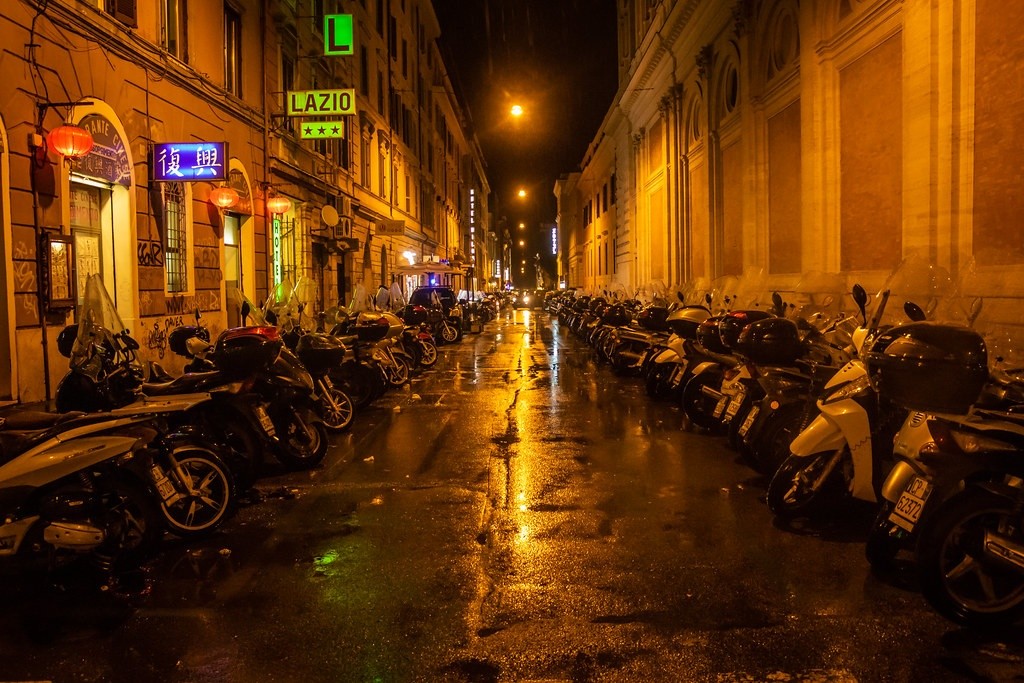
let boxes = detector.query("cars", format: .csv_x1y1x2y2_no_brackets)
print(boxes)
512,289,548,311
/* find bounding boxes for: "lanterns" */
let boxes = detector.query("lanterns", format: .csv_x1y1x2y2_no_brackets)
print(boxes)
46,121,94,160
209,188,240,208
266,197,291,214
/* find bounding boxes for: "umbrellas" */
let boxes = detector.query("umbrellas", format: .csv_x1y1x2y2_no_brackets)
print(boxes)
388,258,465,324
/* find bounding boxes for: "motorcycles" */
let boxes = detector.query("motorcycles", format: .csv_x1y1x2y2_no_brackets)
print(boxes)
0,273,509,591
544,248,1024,640
